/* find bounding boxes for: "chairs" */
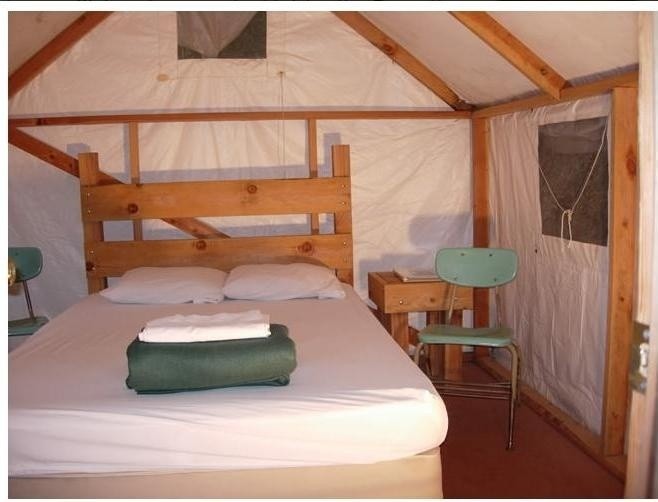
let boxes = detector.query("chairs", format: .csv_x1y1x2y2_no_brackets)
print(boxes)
415,248,520,451
8,247,49,336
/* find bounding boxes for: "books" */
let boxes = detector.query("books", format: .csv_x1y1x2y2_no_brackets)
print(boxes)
393,267,444,283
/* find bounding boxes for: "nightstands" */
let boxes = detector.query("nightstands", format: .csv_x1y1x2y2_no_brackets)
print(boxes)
367,269,474,390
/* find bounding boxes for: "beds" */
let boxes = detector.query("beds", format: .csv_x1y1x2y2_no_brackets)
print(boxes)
9,144,450,499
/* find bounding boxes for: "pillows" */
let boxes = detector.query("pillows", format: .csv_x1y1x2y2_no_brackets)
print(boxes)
225,262,346,301
103,266,225,305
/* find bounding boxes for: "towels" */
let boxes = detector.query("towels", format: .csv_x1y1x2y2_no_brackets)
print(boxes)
140,310,270,343
